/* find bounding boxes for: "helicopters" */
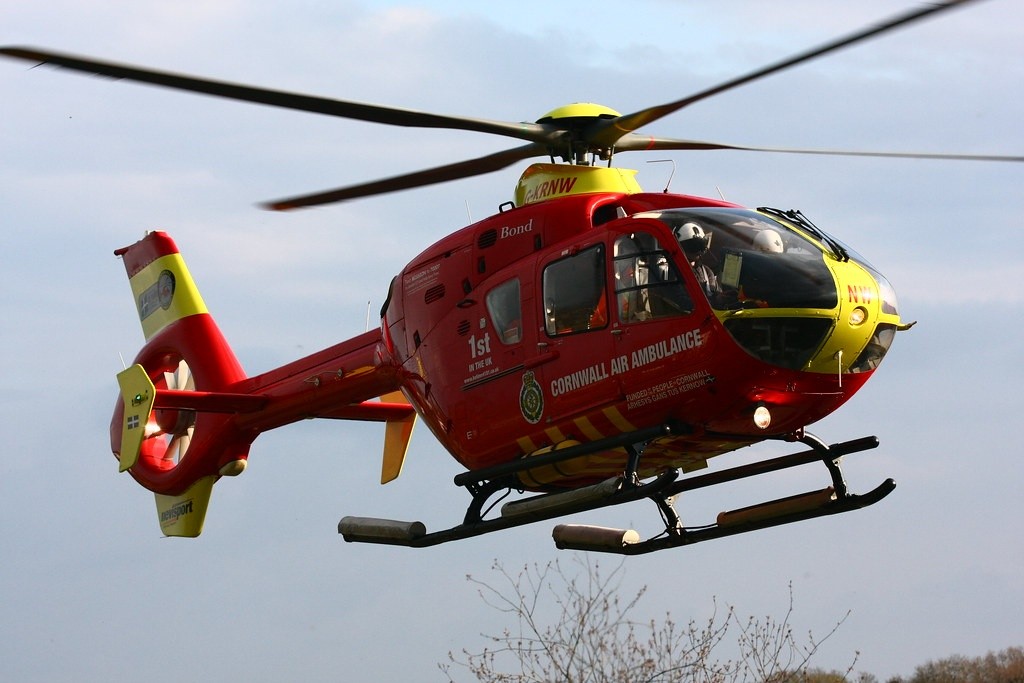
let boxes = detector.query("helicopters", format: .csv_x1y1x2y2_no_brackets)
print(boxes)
1,1,990,563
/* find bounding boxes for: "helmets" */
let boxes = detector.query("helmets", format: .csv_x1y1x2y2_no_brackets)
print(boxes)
751,229,784,253
672,220,708,244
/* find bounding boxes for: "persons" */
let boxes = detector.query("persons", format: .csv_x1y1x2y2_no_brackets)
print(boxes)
657,220,736,316
589,239,643,328
739,229,784,307
